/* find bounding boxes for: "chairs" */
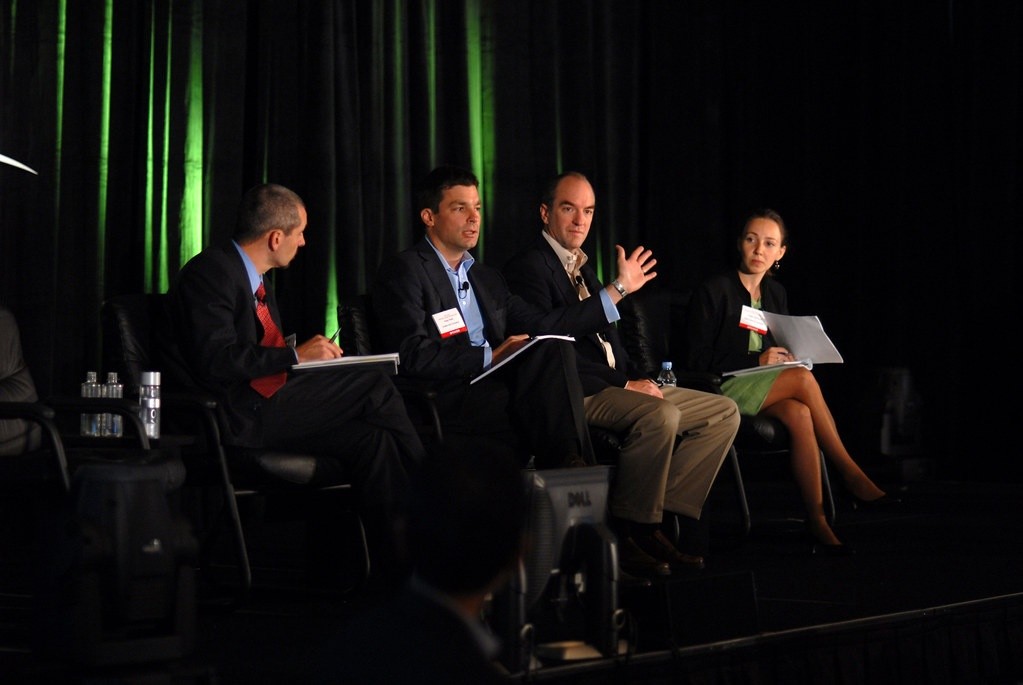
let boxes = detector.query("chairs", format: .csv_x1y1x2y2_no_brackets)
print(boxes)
0,292,837,655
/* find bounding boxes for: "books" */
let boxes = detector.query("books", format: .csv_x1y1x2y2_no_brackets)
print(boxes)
291,352,400,375
469,335,576,384
722,358,813,376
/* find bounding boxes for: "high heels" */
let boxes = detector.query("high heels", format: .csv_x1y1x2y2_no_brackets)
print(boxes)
846,480,905,513
805,523,851,557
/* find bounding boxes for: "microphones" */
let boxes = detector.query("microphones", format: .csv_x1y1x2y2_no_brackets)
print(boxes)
463,281,469,291
262,294,268,305
576,276,583,285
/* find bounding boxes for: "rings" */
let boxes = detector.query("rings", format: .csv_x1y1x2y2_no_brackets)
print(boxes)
777,357,780,361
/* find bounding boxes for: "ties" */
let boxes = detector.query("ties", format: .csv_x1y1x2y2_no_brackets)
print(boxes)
250,282,287,397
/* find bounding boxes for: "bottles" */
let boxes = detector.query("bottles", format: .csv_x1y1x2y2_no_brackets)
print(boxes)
657,362,676,387
100,372,123,437
80,371,100,436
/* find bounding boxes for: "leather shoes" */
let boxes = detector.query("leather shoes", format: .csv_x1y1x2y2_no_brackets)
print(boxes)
631,525,704,571
614,534,671,576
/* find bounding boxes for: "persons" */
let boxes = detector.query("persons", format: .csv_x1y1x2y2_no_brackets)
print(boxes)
376,154,659,562
508,168,742,576
170,179,492,567
685,201,902,560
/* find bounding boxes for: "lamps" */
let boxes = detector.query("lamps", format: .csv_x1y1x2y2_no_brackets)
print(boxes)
877,368,925,496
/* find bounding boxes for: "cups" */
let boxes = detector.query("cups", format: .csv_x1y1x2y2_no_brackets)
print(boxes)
138,371,161,439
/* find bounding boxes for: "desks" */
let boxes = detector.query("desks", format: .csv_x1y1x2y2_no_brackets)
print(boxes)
41,427,199,663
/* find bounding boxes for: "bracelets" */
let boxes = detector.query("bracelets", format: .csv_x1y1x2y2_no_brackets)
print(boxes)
610,279,628,297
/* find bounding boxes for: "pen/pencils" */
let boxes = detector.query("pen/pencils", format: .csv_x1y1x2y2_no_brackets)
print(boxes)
329,328,342,344
778,352,786,355
525,337,536,342
